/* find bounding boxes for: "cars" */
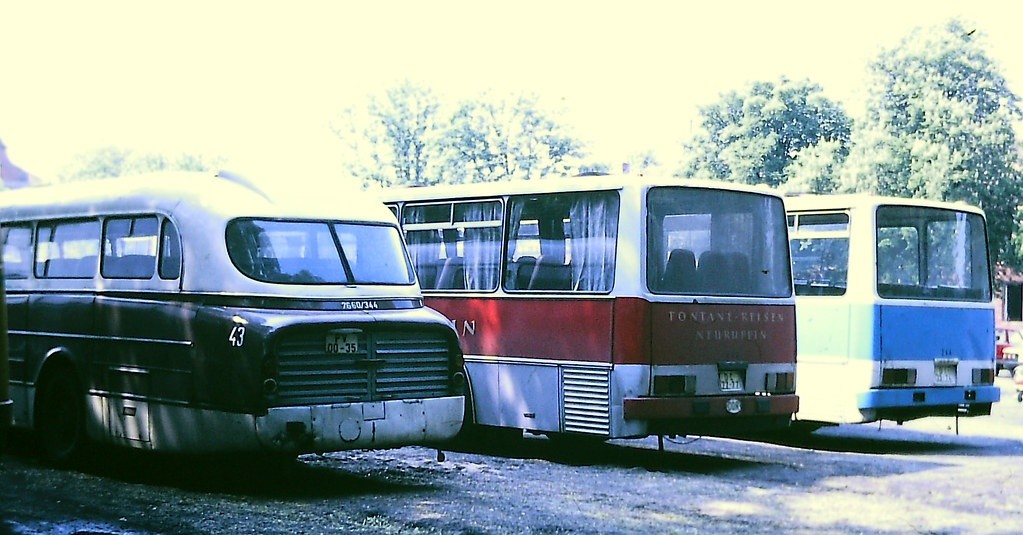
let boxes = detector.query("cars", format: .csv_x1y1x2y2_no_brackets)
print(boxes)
995,326,1023,377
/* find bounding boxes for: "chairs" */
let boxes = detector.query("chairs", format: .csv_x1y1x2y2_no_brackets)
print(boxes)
416,248,573,290
1,254,359,285
656,250,753,294
792,279,846,296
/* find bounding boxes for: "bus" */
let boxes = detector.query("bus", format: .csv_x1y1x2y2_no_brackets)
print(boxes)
784,193,1001,437
0,166,467,495
375,171,800,462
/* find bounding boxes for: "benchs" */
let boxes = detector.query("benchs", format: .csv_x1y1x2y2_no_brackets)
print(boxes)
878,281,983,300
